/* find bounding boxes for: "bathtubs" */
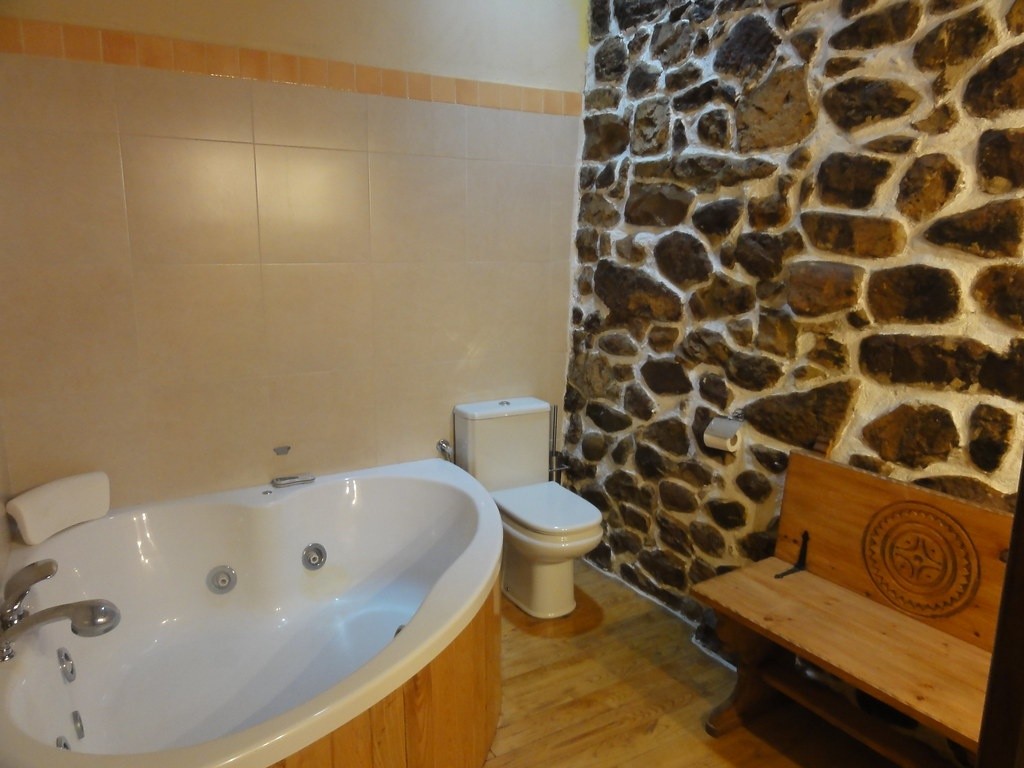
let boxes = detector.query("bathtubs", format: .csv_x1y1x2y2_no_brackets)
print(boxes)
1,456,504,768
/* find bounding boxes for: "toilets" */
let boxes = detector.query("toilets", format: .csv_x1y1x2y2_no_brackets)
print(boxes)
453,396,603,618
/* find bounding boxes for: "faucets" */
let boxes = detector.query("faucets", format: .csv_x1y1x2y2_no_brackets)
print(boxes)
0,558,123,664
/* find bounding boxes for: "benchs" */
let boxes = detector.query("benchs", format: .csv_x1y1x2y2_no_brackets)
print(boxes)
688,446,1014,768
